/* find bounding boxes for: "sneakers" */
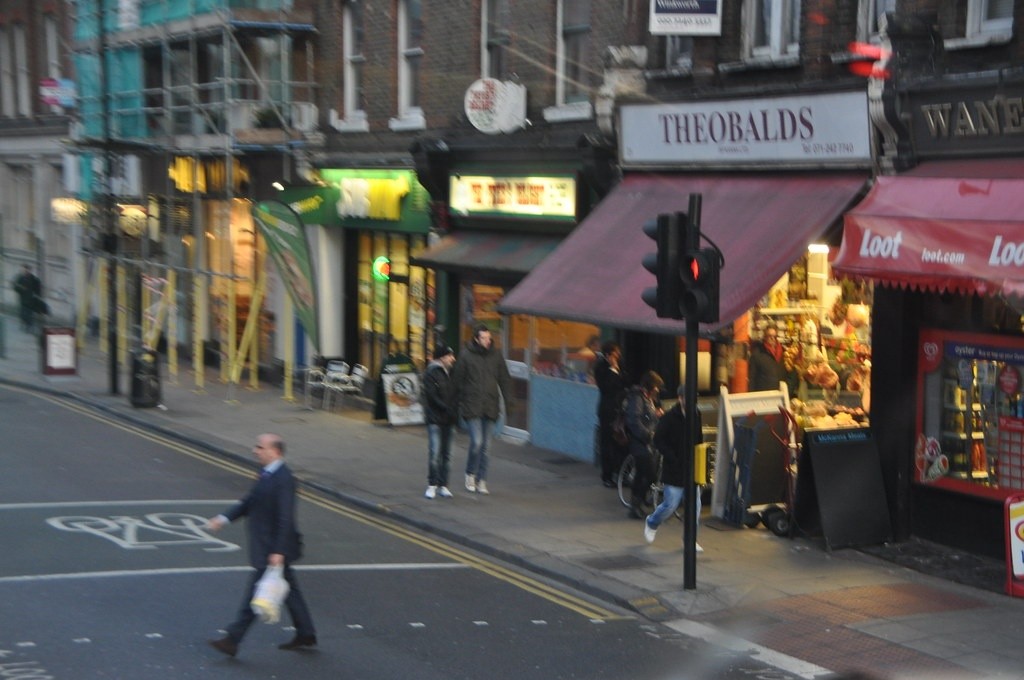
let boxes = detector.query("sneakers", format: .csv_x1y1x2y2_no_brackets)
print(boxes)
696,543,702,551
465,473,476,492
477,480,489,494
425,485,453,499
645,515,657,543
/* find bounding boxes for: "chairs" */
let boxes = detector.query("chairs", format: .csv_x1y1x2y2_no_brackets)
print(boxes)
305,359,350,411
323,363,369,411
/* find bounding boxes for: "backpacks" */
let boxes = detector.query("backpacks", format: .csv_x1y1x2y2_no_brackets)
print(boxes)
612,393,649,447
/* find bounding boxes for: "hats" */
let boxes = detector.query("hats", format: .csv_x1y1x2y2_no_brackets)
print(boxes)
433,345,454,360
677,384,699,396
640,370,663,393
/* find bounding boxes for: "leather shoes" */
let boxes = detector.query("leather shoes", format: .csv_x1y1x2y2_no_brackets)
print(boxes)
279,632,318,650
211,638,238,656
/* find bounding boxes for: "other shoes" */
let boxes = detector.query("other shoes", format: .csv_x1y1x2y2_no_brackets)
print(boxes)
629,506,647,518
604,479,617,487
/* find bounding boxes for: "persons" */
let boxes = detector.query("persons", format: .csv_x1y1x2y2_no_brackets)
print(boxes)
625,371,665,521
594,341,626,489
445,326,515,494
422,344,457,498
206,433,319,657
576,334,600,356
12,263,41,335
645,382,704,554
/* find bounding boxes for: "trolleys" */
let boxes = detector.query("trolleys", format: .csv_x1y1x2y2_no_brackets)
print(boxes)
722,405,803,542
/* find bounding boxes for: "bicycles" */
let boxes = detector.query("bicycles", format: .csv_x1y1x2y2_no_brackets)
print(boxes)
617,436,688,522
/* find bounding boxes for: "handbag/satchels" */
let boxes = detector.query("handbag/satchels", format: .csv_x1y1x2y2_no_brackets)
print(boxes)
293,532,305,561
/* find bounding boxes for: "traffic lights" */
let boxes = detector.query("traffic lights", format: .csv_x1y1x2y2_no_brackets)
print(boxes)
640,211,688,317
678,250,718,322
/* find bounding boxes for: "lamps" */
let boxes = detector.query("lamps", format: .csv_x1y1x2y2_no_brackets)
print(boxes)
808,240,831,254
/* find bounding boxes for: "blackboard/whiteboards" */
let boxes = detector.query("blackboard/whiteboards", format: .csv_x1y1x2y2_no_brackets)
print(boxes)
711,380,797,519
788,427,894,552
44,326,79,374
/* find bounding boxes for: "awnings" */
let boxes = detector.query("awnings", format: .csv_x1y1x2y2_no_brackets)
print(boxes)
835,156,1022,298
495,170,868,338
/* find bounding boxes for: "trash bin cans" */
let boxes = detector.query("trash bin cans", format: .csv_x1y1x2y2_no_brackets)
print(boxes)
128,349,160,407
